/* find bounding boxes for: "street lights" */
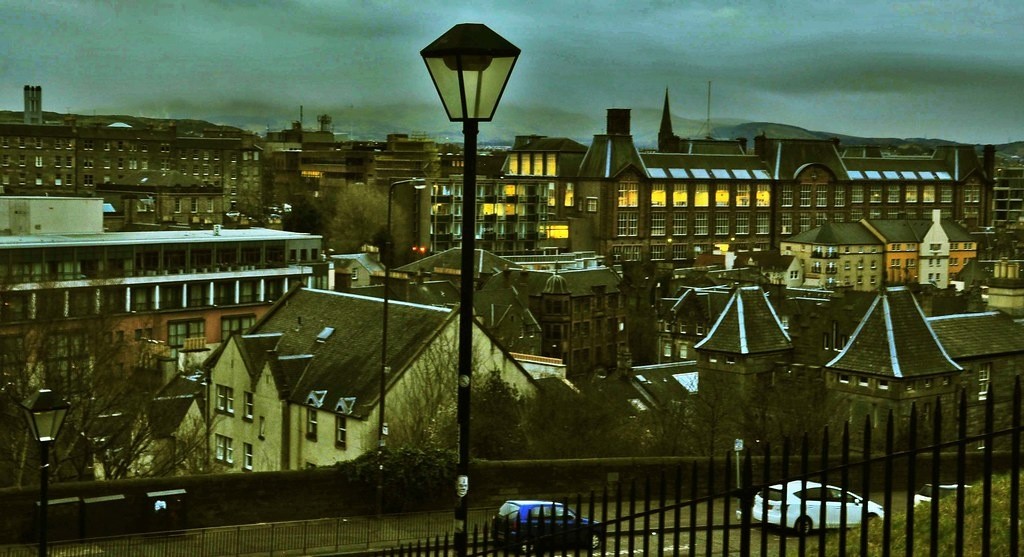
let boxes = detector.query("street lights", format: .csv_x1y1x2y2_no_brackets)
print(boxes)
421,23,523,557
21,388,74,556
377,176,427,453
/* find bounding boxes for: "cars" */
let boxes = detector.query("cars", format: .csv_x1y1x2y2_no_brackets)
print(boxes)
491,500,606,555
751,479,884,534
914,484,973,510
